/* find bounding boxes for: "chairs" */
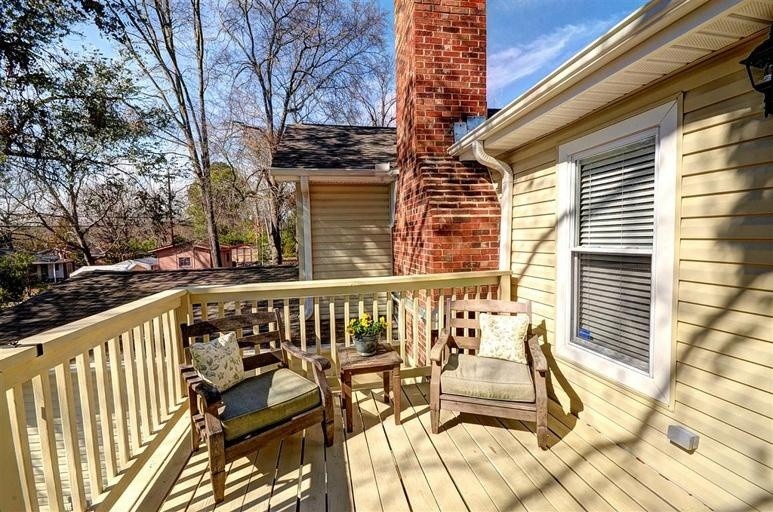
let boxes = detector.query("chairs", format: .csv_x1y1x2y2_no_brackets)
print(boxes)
427,296,550,450
179,308,338,504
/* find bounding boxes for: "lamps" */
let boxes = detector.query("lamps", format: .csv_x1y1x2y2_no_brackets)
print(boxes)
736,30,773,120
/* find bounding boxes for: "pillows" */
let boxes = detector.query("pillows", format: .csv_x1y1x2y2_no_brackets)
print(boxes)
186,331,248,393
475,312,530,365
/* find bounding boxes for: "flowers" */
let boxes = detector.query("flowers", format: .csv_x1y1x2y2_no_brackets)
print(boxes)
346,313,389,337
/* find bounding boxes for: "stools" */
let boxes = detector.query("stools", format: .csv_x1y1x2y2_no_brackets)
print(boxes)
336,342,403,435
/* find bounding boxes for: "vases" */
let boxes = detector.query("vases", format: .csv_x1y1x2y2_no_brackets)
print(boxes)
352,334,378,357
